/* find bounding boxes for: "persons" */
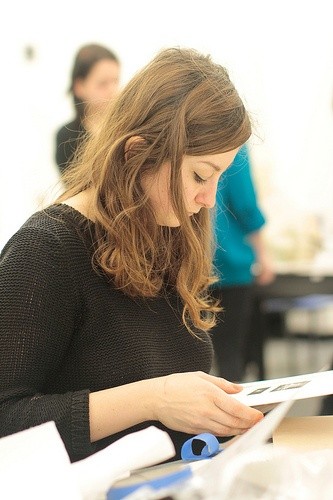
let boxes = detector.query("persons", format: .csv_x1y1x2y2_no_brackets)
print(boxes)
55,44,273,382
0,47,265,462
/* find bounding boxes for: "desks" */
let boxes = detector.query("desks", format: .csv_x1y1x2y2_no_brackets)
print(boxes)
257,274,333,416
